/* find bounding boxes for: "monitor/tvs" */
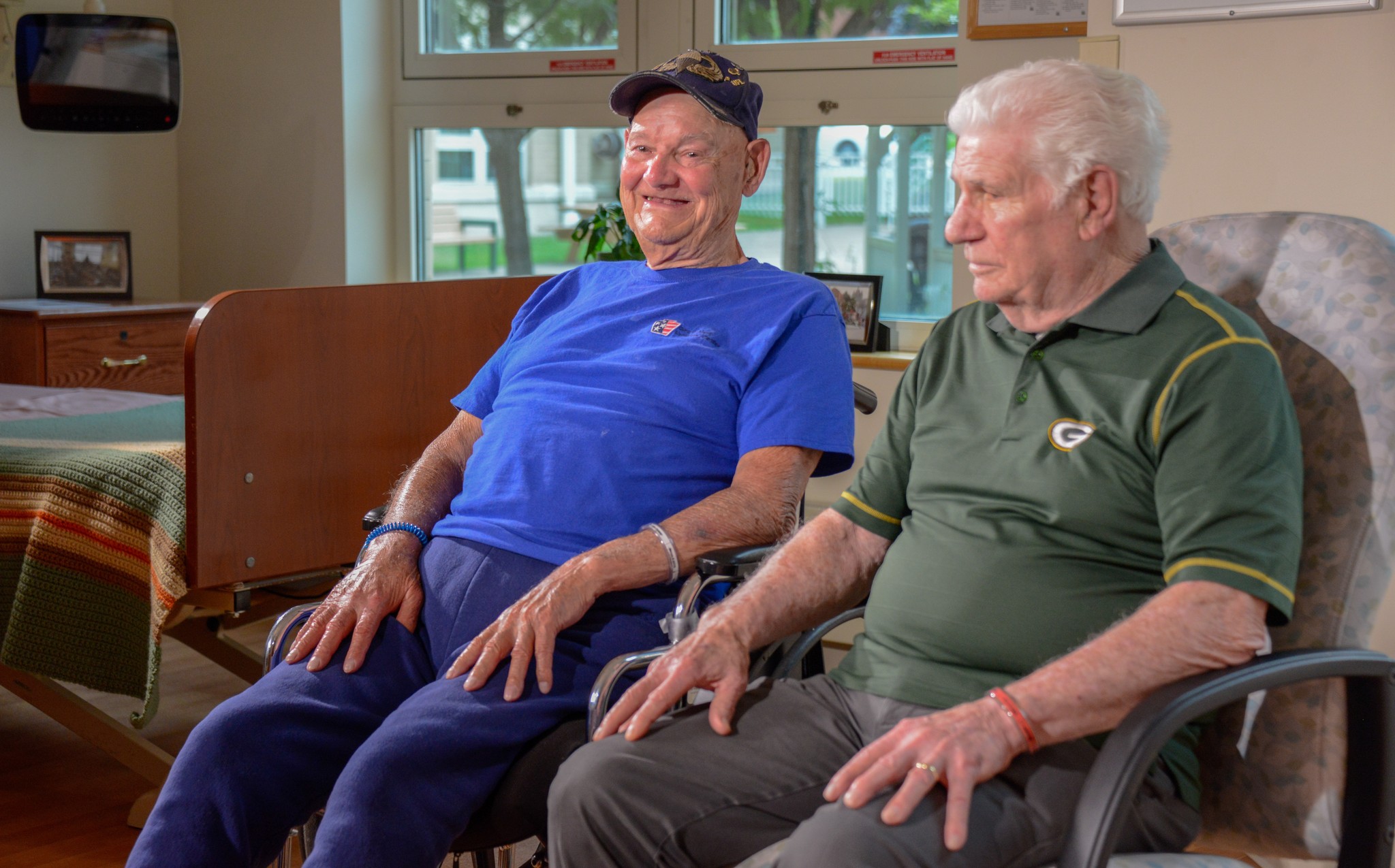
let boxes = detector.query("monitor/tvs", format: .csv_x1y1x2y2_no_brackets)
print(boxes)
16,13,181,134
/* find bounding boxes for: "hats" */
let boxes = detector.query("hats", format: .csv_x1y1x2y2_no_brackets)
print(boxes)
608,50,765,140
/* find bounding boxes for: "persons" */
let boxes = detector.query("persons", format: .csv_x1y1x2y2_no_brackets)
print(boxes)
546,58,1304,868
124,47,857,868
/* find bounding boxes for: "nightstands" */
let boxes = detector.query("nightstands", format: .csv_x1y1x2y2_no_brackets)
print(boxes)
0,300,204,396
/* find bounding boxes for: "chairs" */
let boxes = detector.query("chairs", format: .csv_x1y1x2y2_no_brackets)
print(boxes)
767,212,1395,868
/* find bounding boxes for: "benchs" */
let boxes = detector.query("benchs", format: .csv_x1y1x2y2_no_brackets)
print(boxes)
539,227,615,242
432,205,500,273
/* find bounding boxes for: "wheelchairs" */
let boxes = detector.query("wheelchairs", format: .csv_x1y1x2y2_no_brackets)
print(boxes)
266,380,878,867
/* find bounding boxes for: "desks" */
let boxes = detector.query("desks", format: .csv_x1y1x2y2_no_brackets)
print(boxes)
558,202,610,263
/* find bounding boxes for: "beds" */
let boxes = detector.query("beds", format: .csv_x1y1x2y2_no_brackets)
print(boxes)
0,275,549,829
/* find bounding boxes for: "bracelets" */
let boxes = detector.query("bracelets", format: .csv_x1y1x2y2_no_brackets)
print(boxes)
366,518,429,550
986,687,1041,755
640,523,680,586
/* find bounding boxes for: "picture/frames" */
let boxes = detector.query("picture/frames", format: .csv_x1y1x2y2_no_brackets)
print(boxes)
34,231,132,301
804,272,884,352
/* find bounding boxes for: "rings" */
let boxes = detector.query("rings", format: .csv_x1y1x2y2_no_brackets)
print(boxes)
916,760,940,784
